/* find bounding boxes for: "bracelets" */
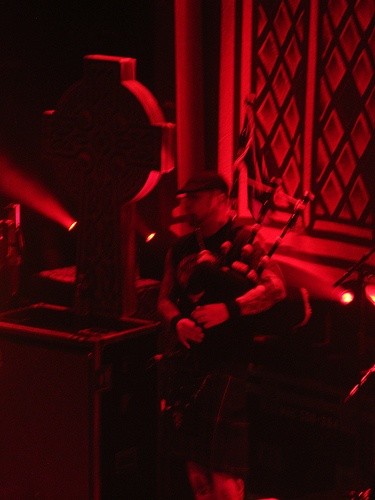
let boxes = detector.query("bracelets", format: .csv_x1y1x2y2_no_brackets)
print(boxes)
223,299,243,321
171,314,187,332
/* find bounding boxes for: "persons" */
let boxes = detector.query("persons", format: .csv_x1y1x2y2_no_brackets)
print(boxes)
154,169,286,455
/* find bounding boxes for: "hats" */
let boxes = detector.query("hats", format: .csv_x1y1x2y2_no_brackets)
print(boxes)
176,172,228,194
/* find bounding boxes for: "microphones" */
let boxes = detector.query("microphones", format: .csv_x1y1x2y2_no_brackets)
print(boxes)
188,218,205,228
